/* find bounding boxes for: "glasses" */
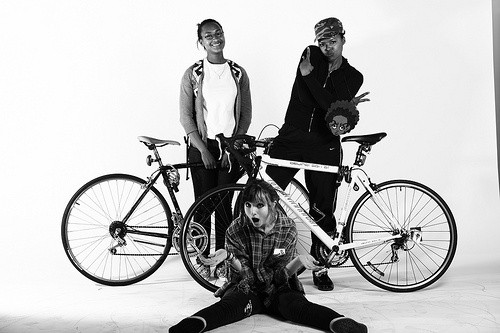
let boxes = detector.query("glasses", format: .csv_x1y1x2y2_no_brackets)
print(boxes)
201,31,225,41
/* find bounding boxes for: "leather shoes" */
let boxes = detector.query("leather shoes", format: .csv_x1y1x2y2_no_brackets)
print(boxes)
313,272,333,290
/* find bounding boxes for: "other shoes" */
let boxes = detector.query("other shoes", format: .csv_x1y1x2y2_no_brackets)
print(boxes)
197,264,227,281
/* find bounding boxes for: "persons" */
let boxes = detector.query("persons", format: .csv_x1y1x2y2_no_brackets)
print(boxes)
263,18,370,289
169,178,368,333
180,19,253,282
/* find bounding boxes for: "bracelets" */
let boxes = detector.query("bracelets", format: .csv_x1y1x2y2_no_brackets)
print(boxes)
225,251,238,264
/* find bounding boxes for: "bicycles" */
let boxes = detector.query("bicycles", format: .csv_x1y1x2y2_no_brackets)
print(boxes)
182,130,457,296
60,134,312,288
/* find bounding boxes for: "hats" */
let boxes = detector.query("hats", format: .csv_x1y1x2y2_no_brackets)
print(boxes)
312,17,345,42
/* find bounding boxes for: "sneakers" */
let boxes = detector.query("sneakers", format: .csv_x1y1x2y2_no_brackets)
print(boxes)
330,317,367,333
168,316,207,333
